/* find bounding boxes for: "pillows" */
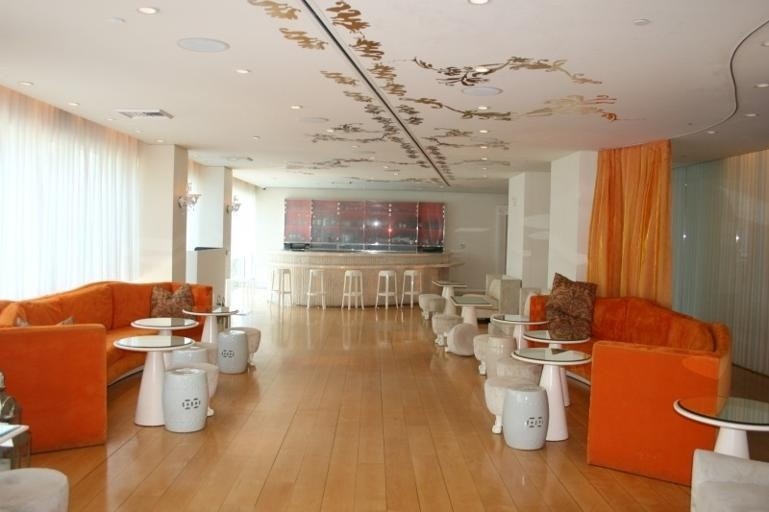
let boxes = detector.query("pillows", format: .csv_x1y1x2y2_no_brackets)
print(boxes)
541,271,600,323
147,283,199,323
12,312,76,329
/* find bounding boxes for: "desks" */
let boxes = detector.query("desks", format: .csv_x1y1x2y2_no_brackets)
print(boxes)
178,304,240,368
111,333,198,428
0,419,35,471
670,391,769,461
263,242,466,308
129,315,201,338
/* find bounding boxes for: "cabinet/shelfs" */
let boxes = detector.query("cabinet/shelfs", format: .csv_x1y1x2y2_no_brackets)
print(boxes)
283,198,447,253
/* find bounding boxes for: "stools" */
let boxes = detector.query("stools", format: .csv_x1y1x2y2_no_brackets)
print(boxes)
268,268,294,311
304,266,330,310
160,325,263,435
1,466,71,511
372,268,400,311
341,269,366,312
414,274,596,451
402,267,423,310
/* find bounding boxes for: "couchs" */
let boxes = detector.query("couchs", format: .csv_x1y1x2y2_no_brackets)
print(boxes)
524,291,734,493
0,279,217,461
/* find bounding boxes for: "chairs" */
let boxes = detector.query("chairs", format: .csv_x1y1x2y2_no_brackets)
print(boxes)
688,444,769,511
